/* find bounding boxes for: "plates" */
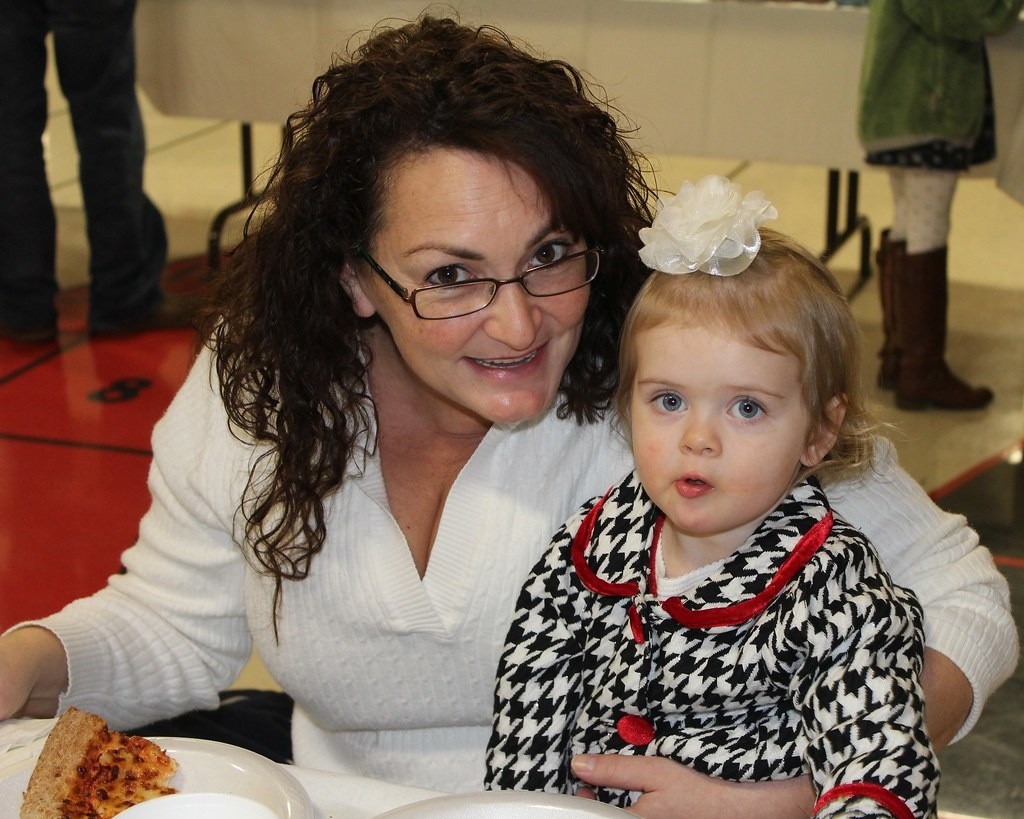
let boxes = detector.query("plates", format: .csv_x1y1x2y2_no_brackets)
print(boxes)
0,736,315,819
110,792,278,819
372,790,643,819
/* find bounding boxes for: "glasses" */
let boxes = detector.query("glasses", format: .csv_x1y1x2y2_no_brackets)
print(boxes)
356,232,606,320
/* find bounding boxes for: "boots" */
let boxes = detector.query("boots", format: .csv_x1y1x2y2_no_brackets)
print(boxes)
877,228,909,391
896,247,993,412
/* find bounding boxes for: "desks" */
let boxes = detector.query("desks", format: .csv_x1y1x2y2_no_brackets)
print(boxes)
135,1,1023,291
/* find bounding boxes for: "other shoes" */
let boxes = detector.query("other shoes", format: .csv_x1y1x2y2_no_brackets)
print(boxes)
0,317,57,340
88,295,192,334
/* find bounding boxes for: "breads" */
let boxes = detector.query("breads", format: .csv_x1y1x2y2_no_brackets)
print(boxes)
20,706,180,819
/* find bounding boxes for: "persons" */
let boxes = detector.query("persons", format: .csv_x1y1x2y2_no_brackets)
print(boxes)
1,7,1019,819
0,0,169,346
483,174,943,819
853,0,1024,410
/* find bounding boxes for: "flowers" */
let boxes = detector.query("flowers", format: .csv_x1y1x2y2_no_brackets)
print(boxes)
638,171,780,275
